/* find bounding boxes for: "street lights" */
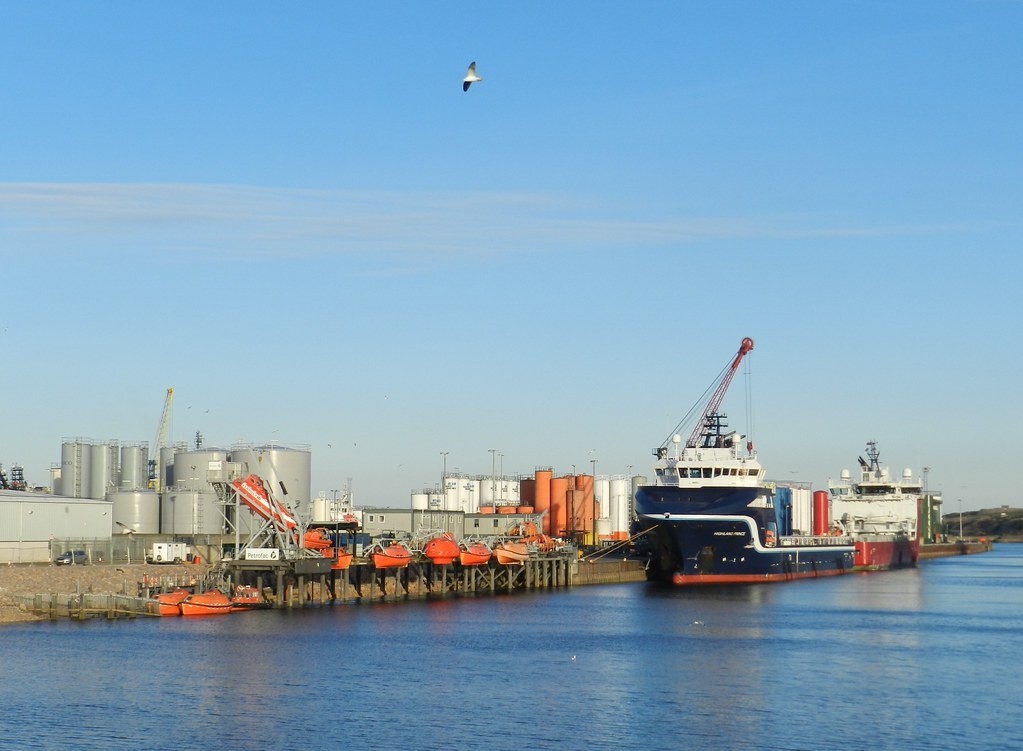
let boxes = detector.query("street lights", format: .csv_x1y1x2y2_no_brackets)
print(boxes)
957,497,962,540
573,465,576,475
440,451,450,510
488,449,498,512
591,460,598,476
627,465,632,475
497,454,504,505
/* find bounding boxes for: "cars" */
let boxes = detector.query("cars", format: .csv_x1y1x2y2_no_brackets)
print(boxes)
53,551,88,566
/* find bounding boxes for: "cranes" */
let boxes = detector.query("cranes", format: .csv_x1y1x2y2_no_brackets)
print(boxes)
148,387,174,489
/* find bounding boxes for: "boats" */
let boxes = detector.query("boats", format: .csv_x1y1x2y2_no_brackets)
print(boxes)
147,589,189,617
314,547,353,571
478,505,496,514
228,587,264,612
232,475,295,533
516,505,534,514
496,506,516,514
628,410,858,589
291,527,332,549
492,541,530,566
178,588,233,614
458,542,492,565
828,438,925,571
369,541,411,568
425,533,460,565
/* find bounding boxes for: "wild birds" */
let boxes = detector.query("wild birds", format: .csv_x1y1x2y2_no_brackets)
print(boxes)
462,61,483,92
